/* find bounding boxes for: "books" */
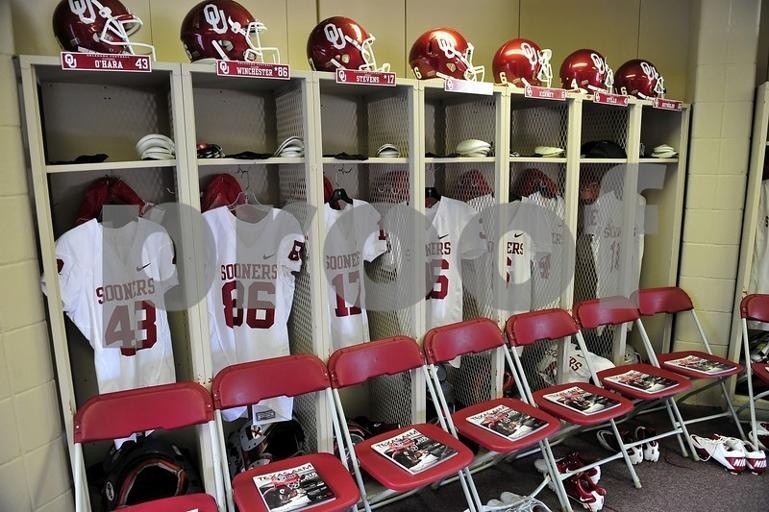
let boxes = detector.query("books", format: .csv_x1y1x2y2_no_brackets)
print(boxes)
465,404,549,442
664,354,738,375
543,385,622,417
604,369,680,394
371,428,459,476
252,461,336,511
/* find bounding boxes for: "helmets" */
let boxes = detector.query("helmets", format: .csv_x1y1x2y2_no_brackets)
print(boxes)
180,1,280,63
493,38,554,88
306,15,393,73
409,29,487,81
53,1,157,62
614,58,667,99
560,49,616,95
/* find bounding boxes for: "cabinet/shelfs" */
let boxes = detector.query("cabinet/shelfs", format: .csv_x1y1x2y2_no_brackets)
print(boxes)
310,69,423,451
722,82,768,410
620,97,696,363
182,61,327,498
18,55,217,511
503,84,570,394
417,76,502,425
563,91,637,382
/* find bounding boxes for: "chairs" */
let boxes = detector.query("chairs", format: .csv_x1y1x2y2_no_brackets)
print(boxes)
506,305,642,512
211,353,362,512
425,318,573,512
328,336,482,512
730,292,768,459
574,296,700,489
71,379,226,512
630,284,748,462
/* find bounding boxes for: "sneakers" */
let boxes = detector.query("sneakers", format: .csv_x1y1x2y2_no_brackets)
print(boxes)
690,434,746,475
543,468,606,512
747,431,769,452
486,498,521,512
500,491,552,512
596,429,643,465
749,420,769,432
635,426,660,462
534,448,601,485
712,433,767,476
